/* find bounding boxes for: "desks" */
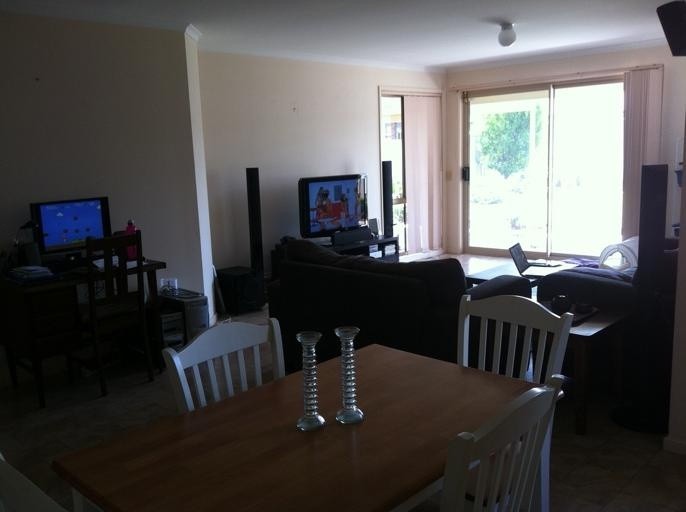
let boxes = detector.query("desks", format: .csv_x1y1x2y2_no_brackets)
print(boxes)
0,258,168,409
48,342,568,511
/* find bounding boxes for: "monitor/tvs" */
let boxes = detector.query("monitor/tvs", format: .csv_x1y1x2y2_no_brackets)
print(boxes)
299,174,358,238
30,196,113,252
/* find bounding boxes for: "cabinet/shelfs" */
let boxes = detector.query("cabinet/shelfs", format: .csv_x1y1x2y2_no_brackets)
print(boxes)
321,236,400,265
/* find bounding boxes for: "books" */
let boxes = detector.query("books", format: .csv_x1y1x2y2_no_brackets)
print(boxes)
10,265,54,280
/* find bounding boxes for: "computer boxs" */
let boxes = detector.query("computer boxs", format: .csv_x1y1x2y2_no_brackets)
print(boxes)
161,288,208,345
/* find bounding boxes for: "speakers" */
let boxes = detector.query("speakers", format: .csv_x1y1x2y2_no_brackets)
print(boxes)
217,267,264,315
382,160,394,237
21,243,42,266
246,169,264,269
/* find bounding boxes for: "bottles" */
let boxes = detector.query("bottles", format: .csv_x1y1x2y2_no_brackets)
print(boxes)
124,219,139,260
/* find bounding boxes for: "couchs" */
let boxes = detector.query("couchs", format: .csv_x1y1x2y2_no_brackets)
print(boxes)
535,238,680,439
266,235,533,381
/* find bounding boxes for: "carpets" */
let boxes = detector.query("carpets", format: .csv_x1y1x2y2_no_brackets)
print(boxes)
0,322,664,511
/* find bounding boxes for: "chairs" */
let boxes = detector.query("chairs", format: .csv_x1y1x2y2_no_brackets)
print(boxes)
456,290,575,511
68,229,158,396
161,317,289,415
437,372,565,512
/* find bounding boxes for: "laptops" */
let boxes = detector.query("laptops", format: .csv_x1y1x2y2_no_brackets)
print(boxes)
510,244,550,278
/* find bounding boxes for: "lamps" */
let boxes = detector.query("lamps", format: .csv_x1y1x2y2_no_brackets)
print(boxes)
11,218,40,262
496,23,518,49
